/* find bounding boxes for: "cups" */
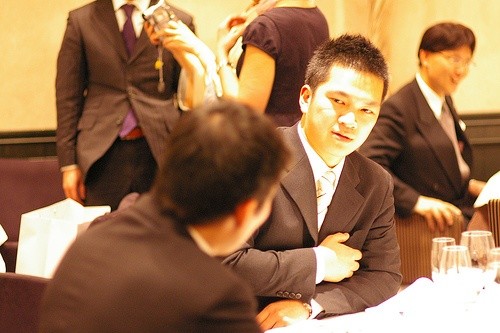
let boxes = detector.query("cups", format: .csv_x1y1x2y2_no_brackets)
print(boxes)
431,231,500,296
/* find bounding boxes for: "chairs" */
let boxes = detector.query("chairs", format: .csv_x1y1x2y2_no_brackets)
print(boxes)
0,155,61,272
395,212,464,289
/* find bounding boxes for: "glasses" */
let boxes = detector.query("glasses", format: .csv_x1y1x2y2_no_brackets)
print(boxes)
443,53,475,68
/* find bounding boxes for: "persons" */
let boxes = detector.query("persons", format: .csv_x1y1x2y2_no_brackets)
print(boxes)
143,17,247,111
54,0,206,212
214,0,329,130
358,23,488,234
37,96,294,333
214,35,402,331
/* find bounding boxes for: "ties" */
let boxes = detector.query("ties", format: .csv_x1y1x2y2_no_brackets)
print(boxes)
443,103,470,179
119,4,138,139
315,171,335,233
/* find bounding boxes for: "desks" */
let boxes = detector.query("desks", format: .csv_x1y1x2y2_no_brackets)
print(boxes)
262,266,500,333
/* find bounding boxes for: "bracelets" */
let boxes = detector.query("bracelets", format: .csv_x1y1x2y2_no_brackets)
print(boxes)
216,61,233,74
300,302,312,320
